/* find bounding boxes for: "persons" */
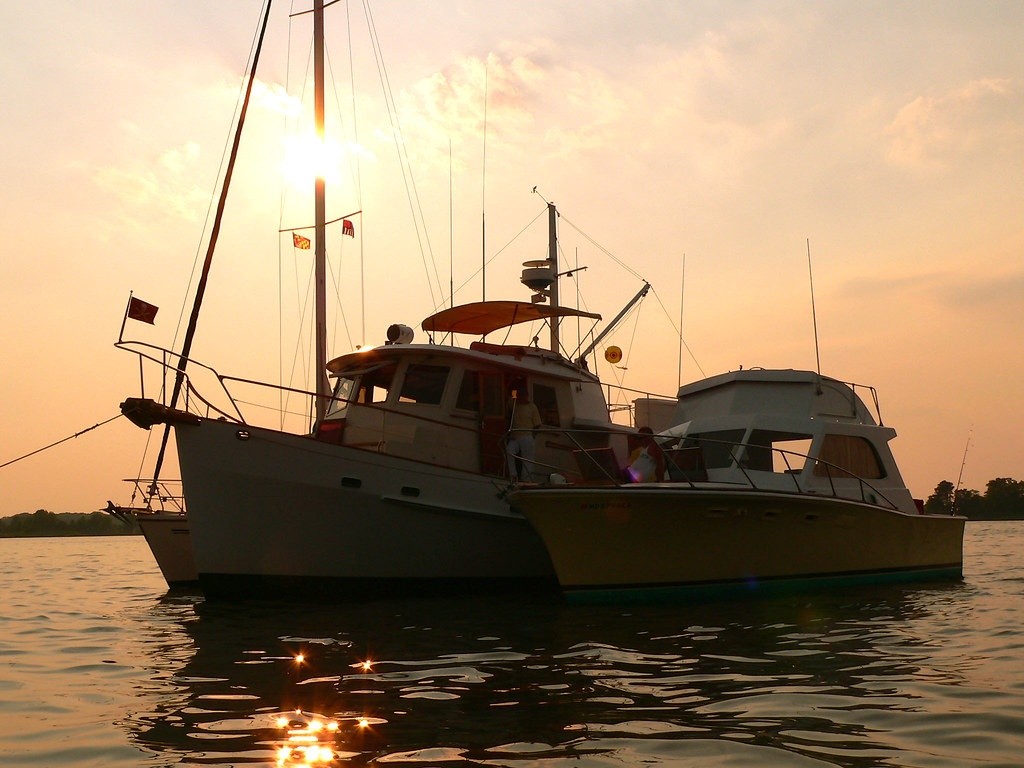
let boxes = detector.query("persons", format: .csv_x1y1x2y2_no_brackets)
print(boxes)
507,387,542,482
626,427,665,482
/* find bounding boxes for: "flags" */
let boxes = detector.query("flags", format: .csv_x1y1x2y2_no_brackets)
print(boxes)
128,297,159,325
293,233,310,250
342,219,354,239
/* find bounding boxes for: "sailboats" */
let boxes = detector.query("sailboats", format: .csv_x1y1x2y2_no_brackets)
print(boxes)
104,1,968,608
